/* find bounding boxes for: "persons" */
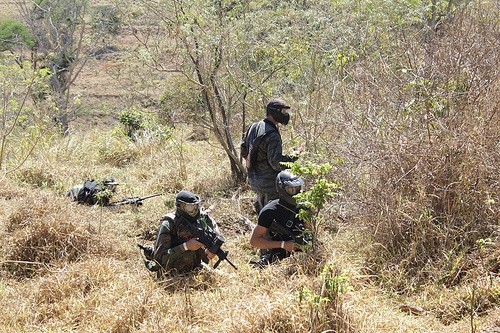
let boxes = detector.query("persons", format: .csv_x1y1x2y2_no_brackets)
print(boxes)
67,175,120,207
239,100,304,214
250,171,313,271
156,190,226,278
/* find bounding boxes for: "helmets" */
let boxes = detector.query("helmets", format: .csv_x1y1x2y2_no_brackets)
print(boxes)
176,192,201,221
267,100,291,126
276,170,304,206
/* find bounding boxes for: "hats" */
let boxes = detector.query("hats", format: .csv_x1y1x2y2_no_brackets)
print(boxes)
102,176,119,186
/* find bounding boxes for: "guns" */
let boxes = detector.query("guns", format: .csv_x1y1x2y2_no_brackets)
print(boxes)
267,218,312,251
176,213,239,272
106,193,162,208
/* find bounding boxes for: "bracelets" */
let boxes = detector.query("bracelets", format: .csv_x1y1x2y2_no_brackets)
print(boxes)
281,240,285,248
